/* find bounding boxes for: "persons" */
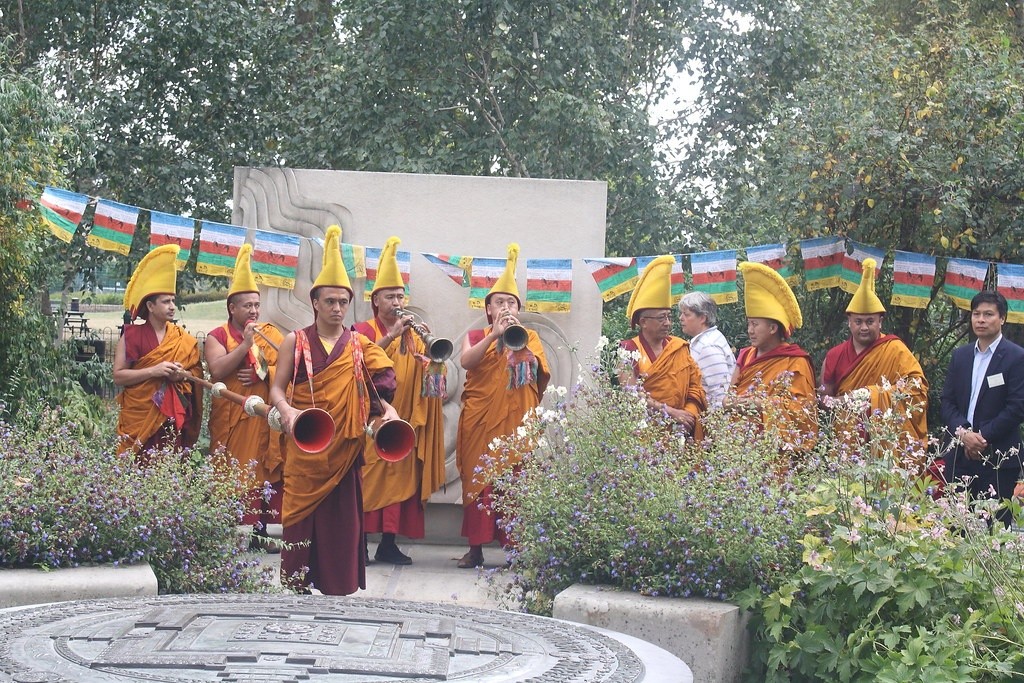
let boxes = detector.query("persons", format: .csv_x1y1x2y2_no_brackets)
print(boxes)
350,273,448,564
818,295,930,502
940,290,1024,546
202,281,287,554
113,280,205,468
722,299,819,485
455,277,551,568
679,292,738,413
616,290,709,452
271,266,401,597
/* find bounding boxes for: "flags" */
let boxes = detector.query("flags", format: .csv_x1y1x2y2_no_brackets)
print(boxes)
12,178,1024,324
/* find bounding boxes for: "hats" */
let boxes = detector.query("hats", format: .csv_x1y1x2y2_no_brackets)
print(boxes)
310,224,354,320
844,257,887,313
738,260,803,340
484,242,522,324
123,243,181,321
227,242,260,317
371,235,406,316
626,254,677,330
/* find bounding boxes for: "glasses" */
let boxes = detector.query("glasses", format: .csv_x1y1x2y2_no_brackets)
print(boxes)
640,313,673,322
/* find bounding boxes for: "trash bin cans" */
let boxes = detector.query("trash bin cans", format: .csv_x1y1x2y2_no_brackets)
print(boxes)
75,338,106,364
71,298,79,311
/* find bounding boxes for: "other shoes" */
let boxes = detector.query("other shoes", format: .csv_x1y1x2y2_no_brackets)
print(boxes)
374,542,413,565
248,529,281,553
457,551,485,568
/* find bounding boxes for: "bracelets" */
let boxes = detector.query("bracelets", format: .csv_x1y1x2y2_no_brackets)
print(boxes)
184,370,188,381
387,332,395,341
276,398,285,408
